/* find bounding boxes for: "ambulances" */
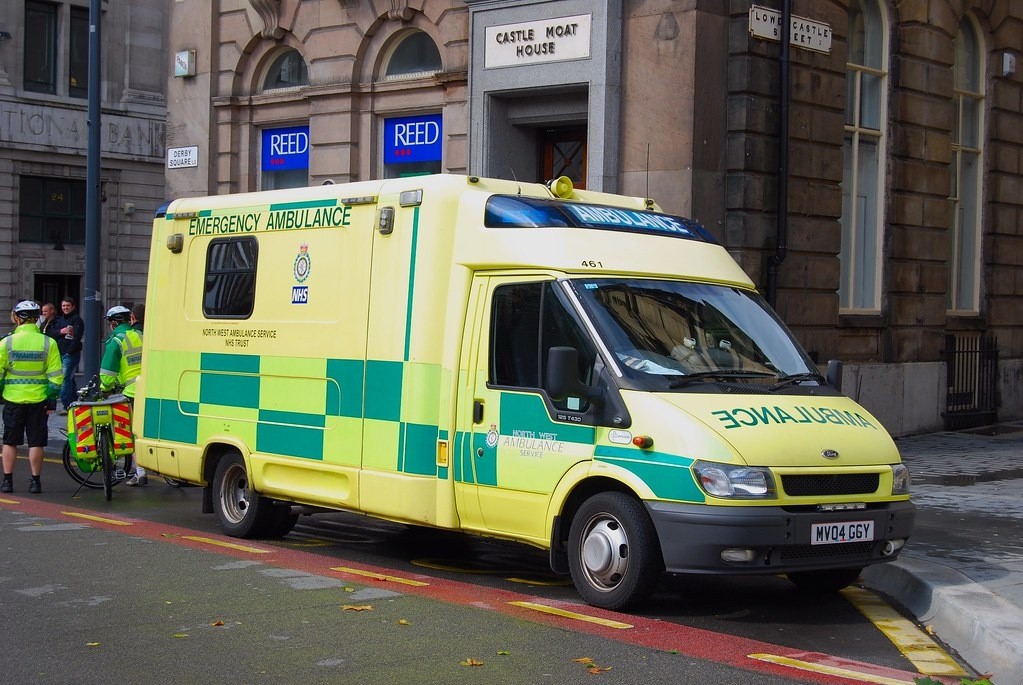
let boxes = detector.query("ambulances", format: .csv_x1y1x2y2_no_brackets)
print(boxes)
132,174,917,613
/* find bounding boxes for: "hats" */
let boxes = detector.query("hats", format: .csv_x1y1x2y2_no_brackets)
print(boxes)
132,304,144,320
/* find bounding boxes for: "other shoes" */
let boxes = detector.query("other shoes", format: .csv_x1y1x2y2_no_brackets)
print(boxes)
59,410,68,416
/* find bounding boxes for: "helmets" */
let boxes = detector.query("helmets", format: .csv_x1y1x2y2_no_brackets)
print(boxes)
103,306,131,321
15,300,41,317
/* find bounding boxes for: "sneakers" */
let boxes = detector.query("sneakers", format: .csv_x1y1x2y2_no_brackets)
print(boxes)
1,479,13,492
29,479,41,493
127,473,147,485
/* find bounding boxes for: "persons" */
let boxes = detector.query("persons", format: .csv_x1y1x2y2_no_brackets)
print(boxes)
52,297,84,414
39,303,58,335
98,302,148,486
0,301,65,494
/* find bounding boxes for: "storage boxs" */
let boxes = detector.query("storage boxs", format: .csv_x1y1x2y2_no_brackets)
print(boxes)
67,394,133,474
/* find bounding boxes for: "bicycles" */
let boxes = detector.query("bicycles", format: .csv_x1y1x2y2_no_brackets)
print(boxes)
60,375,132,502
